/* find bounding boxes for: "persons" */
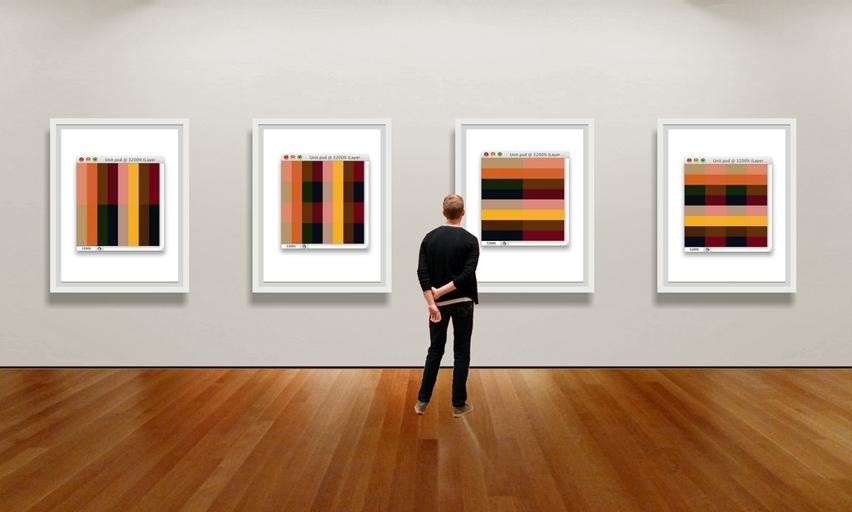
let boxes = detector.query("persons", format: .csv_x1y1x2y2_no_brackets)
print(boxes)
412,195,479,417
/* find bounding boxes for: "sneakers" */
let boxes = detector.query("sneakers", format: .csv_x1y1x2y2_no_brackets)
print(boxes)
452,402,474,418
414,400,427,415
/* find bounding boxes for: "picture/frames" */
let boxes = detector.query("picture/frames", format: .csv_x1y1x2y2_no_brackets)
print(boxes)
251,118,392,296
48,117,190,294
455,118,594,295
656,119,797,295
